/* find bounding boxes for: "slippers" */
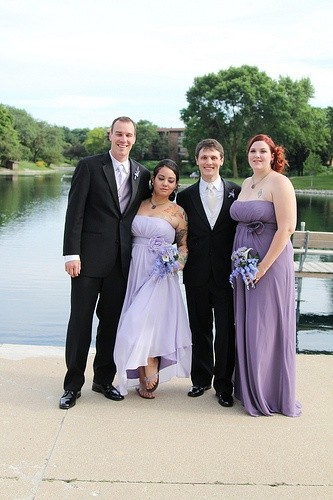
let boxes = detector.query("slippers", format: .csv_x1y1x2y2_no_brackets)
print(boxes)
135,385,154,399
143,356,161,392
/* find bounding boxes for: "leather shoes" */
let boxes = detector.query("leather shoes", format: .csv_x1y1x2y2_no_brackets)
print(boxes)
92,382,123,400
216,391,233,407
187,384,211,397
59,389,81,409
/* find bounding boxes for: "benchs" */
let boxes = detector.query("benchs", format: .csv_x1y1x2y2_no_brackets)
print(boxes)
291,230,333,270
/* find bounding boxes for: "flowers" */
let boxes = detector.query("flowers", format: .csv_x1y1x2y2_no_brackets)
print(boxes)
153,243,185,278
229,246,259,290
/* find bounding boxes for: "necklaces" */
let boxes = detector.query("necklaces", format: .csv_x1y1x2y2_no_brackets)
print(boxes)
251,172,271,189
149,198,163,209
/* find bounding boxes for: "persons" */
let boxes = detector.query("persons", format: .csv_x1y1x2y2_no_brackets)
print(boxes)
230,134,303,418
58,116,154,409
176,138,242,407
113,159,192,400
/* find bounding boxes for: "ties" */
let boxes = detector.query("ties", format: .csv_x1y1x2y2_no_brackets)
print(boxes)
206,184,215,211
116,166,123,189
